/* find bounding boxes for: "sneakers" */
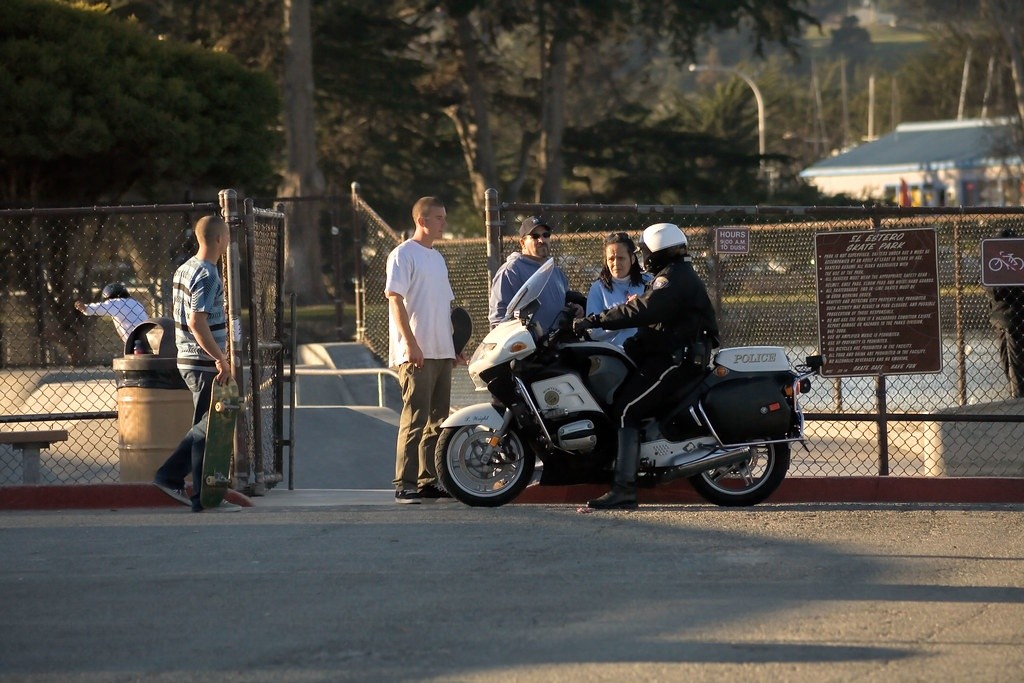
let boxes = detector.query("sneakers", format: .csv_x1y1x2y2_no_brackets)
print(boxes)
394,484,458,503
153,481,193,507
200,499,243,512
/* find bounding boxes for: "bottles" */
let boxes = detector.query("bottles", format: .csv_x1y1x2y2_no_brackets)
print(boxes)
133,340,144,355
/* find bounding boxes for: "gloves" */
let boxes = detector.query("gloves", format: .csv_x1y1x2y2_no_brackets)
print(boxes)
572,314,598,338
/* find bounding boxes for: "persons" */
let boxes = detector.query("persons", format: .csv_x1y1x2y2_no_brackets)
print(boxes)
585,232,655,353
151,215,244,513
488,216,584,349
573,222,722,510
75,283,150,345
985,230,1024,398
386,196,468,503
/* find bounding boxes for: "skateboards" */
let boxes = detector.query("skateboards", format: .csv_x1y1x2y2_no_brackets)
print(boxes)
451,307,472,355
199,373,248,510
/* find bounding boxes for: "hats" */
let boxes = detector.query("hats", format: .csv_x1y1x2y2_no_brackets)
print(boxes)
519,217,552,237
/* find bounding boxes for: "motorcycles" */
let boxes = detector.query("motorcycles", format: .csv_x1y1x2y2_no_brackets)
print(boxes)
434,255,826,510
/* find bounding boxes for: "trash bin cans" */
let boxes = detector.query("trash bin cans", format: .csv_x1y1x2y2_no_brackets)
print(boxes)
113,319,195,482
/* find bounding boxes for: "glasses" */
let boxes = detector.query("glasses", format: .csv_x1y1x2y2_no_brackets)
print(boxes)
529,232,551,240
606,232,629,239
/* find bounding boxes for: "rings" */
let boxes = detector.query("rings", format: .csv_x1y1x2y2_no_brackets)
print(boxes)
415,363,418,367
418,367,420,368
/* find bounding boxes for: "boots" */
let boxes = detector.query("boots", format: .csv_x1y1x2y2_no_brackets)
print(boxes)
586,427,643,509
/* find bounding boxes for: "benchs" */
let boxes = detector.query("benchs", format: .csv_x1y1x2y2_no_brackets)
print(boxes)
0,430,69,484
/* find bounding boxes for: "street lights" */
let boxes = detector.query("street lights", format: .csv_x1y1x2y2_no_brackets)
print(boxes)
688,65,768,169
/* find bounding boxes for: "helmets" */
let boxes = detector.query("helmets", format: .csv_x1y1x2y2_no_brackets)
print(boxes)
632,223,689,274
102,283,131,299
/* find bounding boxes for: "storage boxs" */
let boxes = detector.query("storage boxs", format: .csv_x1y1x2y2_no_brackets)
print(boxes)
707,378,792,441
